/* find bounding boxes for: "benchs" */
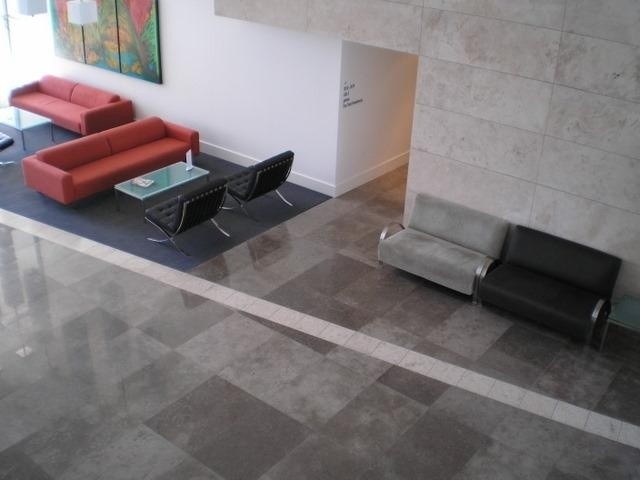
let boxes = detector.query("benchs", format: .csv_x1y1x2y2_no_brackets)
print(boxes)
476,222,623,354
377,193,510,306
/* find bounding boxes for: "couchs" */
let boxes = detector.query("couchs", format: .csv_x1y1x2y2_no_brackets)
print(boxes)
7,73,199,212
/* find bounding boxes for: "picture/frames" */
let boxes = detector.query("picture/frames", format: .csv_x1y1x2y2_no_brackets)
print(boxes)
52,1,165,85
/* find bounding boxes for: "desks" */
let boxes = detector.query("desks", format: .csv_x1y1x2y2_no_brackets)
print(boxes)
0,131,13,151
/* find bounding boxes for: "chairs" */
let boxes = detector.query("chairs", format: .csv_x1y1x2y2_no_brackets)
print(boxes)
146,152,305,258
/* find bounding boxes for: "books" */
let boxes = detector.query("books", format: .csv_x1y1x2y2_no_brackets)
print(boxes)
131,176,154,188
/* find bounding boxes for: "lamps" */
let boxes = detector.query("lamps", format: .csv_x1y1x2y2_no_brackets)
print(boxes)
18,0,48,17
67,0,98,26
